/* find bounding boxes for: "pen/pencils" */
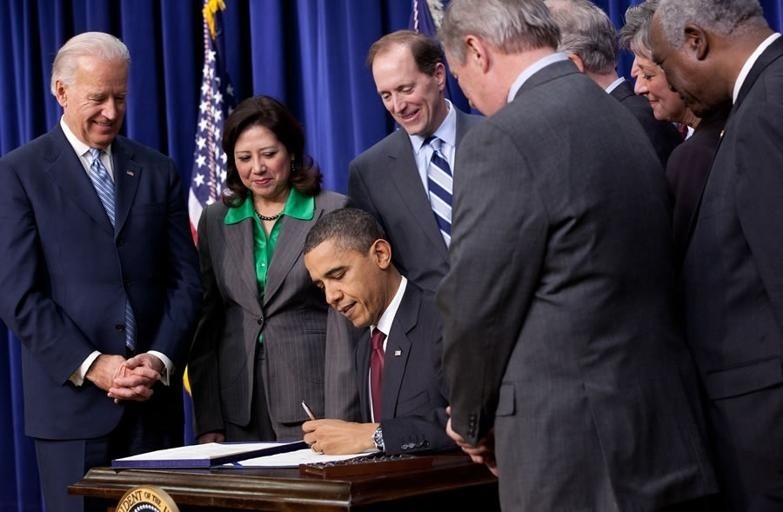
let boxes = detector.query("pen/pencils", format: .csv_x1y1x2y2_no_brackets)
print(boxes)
301,402,315,420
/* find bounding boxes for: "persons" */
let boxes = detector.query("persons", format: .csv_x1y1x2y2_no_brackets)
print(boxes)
618,0,727,241
0,30,202,512
440,0,674,512
543,0,686,169
347,30,486,296
648,0,783,512
187,94,354,444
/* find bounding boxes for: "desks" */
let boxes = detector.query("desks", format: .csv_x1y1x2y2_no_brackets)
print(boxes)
67,464,499,512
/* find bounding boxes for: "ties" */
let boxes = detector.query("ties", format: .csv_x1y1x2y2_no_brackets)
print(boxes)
369,328,385,424
87,145,135,353
421,132,453,248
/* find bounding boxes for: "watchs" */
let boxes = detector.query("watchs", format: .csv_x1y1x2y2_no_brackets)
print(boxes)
373,425,384,450
